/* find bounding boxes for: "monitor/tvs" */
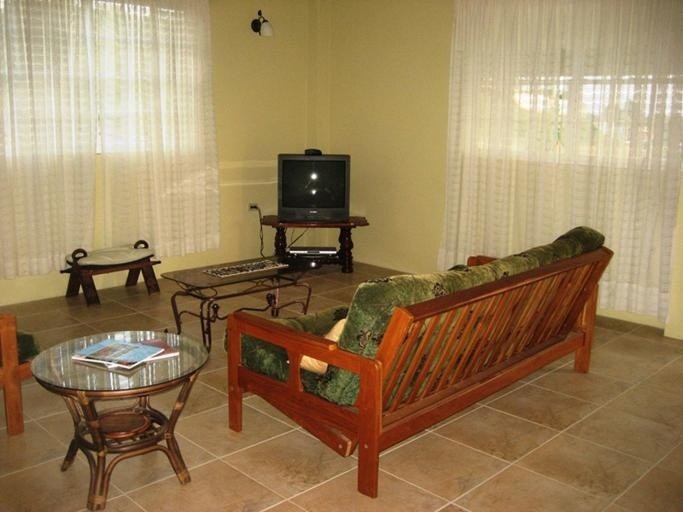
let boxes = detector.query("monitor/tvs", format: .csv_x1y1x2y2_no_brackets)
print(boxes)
277,154,351,223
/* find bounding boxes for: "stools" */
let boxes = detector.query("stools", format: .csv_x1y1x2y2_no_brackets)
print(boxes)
58,240,161,307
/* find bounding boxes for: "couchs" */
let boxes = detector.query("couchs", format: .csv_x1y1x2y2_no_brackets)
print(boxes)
223,226,614,500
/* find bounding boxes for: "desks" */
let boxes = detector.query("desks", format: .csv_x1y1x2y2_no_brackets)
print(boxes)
260,215,369,273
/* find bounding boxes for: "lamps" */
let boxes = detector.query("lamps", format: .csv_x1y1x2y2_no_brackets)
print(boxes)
250,10,273,39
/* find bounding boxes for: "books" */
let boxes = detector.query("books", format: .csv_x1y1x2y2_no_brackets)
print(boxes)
70,337,180,369
70,338,165,371
